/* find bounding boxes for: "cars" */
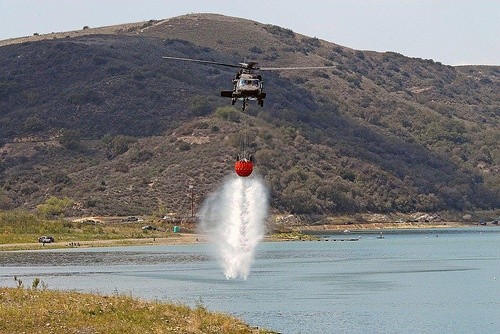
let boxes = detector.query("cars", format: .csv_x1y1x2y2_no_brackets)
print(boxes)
38,236,54,243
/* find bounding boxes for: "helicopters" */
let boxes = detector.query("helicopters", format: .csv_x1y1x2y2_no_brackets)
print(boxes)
161,55,336,111
